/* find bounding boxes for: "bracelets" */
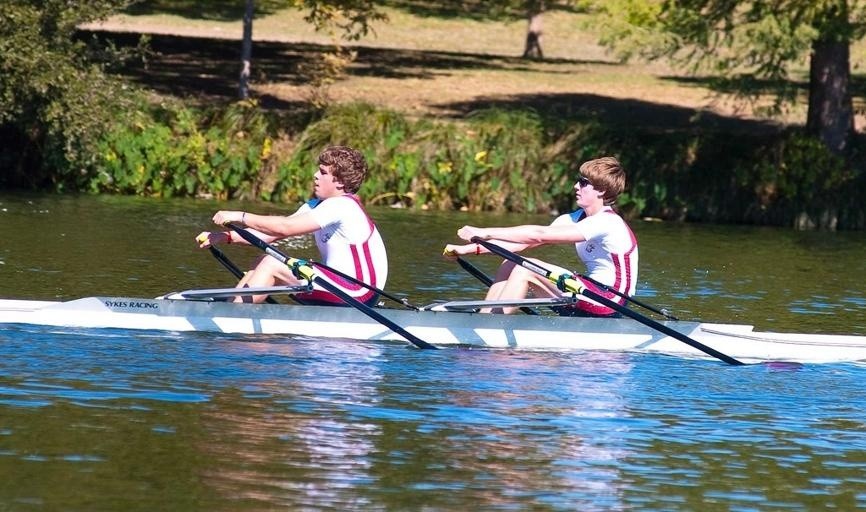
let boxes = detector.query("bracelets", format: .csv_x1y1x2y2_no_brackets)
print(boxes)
488,232,491,240
476,242,480,255
242,211,246,225
228,230,232,244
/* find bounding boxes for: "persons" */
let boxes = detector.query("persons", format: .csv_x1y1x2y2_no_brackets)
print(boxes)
523,13,544,60
444,156,638,316
195,146,389,306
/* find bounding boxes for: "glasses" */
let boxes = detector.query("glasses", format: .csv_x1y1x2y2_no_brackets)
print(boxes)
578,174,592,187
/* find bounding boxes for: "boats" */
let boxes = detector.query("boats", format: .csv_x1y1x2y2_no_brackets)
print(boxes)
0,293,866,368
